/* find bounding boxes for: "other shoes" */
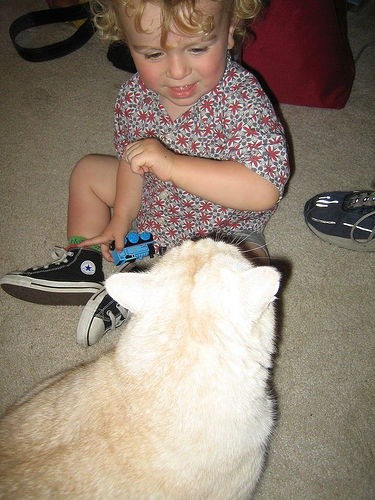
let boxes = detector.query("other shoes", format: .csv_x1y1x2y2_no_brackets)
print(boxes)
106,39,137,74
1,244,106,306
76,261,149,348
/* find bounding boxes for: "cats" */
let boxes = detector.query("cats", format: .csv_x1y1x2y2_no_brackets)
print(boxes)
1,228,283,500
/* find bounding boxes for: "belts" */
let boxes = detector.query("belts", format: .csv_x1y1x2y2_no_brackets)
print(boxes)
9,2,99,63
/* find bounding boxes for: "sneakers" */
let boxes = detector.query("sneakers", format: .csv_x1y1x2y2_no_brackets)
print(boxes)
304,189,375,252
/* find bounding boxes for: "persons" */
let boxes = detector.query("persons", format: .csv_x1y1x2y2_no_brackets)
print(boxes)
1,0,292,348
305,189,374,250
